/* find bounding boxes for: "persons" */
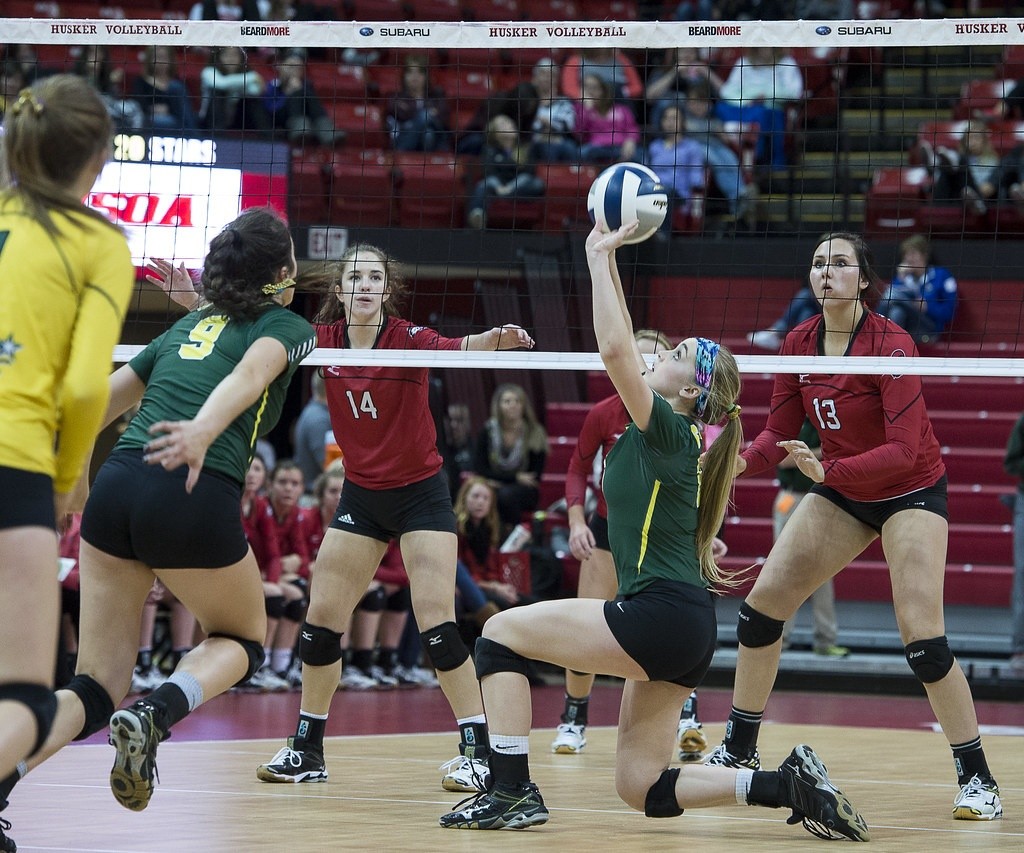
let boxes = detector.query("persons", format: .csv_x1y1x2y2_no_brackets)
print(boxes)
437,222,870,844
0,0,1024,853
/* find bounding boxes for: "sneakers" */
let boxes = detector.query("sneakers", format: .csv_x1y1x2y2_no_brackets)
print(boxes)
0,799,17,853
439,743,490,791
950,772,1004,821
438,774,549,829
702,739,763,771
130,649,440,693
256,735,328,783
677,719,708,761
780,744,870,842
552,712,588,753
108,699,173,812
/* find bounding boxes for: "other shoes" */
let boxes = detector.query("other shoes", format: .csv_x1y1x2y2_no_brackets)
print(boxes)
747,326,782,350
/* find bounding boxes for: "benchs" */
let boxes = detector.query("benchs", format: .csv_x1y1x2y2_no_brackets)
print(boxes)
0,0,1024,239
0,274,1024,611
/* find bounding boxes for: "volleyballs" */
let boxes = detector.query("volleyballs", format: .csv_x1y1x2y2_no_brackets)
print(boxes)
587,162,668,240
